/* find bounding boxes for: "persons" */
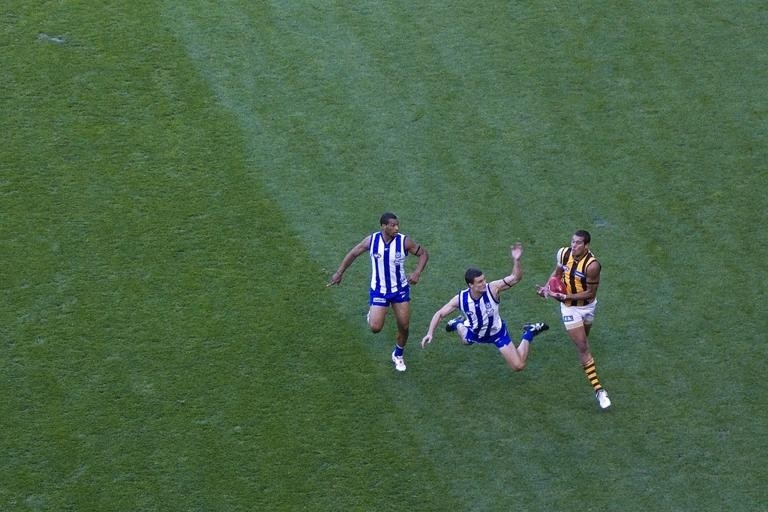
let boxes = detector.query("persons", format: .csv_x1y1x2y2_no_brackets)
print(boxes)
534,228,613,410
421,241,550,372
326,212,429,371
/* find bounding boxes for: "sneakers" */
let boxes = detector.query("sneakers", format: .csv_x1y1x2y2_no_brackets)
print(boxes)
392,351,406,371
446,315,465,332
596,390,611,409
524,322,549,332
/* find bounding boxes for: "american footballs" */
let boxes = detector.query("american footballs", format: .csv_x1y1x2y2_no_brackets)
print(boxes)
549,277,566,293
